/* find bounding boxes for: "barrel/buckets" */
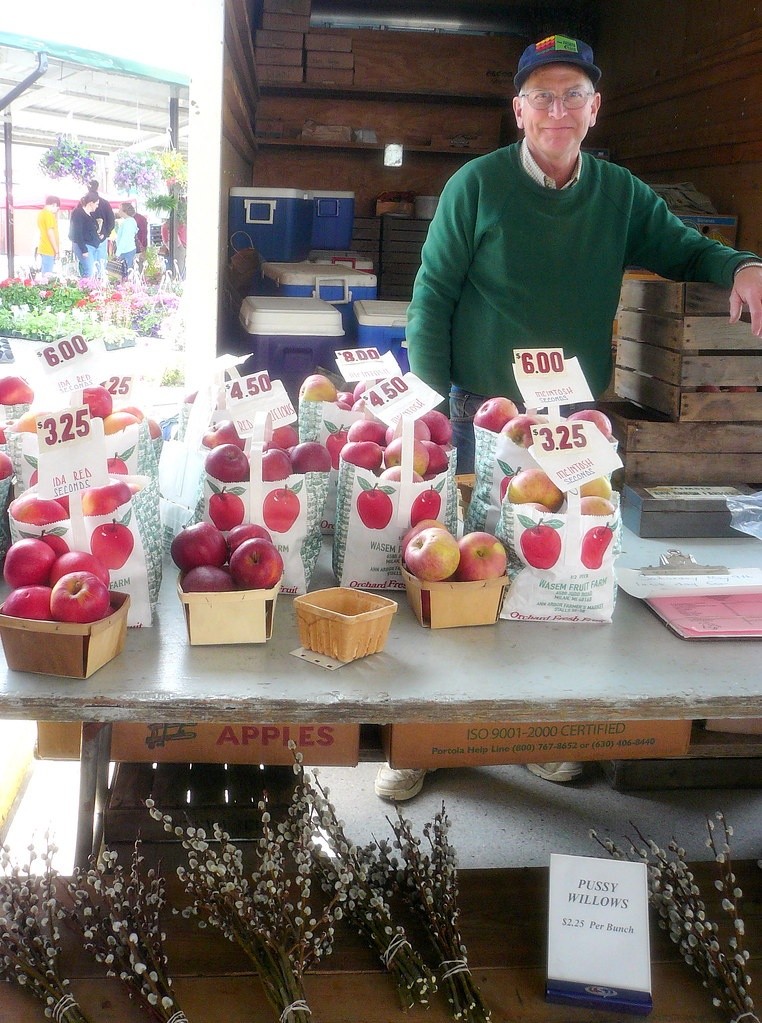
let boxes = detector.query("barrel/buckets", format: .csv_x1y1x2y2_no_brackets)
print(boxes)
414,195,439,219
239,296,345,406
259,263,378,342
350,299,411,375
299,188,356,250
227,186,312,262
308,250,373,276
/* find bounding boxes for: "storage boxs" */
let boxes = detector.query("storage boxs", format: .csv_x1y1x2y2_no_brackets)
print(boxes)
621,482,758,538
380,720,691,770
376,200,414,216
259,262,377,348
304,190,355,249
597,400,762,494
0,590,131,680
228,186,305,260
238,297,346,405
311,250,373,278
353,299,411,377
614,279,762,423
301,125,356,142
672,212,739,250
37,721,359,768
399,557,509,630
254,0,356,85
176,576,283,645
293,587,399,664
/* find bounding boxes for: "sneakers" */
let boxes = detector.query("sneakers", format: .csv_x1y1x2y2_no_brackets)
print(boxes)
376,763,435,802
528,761,582,781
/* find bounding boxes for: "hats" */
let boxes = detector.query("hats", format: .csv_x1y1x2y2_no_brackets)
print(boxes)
513,34,602,91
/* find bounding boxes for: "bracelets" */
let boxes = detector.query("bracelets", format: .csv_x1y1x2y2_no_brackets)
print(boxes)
735,261,762,275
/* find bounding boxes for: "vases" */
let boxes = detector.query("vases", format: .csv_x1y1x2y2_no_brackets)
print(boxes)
102,337,136,351
16,332,25,338
0,330,8,337
9,330,15,337
30,333,40,341
40,332,64,343
26,334,30,339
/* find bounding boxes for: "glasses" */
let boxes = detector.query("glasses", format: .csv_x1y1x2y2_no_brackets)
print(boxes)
518,89,599,110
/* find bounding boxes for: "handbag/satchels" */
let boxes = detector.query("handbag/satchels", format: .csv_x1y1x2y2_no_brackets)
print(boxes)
0,350,622,628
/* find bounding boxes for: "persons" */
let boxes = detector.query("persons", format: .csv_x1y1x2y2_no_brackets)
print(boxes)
37,195,60,277
66,179,149,280
374,32,762,801
161,212,186,279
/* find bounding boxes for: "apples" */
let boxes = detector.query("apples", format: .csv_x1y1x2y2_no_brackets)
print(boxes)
401,519,508,620
0,377,450,524
508,469,617,516
474,397,613,449
171,521,283,592
3,537,117,625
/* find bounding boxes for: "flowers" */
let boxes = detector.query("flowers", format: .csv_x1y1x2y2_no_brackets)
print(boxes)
0,131,190,346
589,809,762,1023
0,741,495,1023
